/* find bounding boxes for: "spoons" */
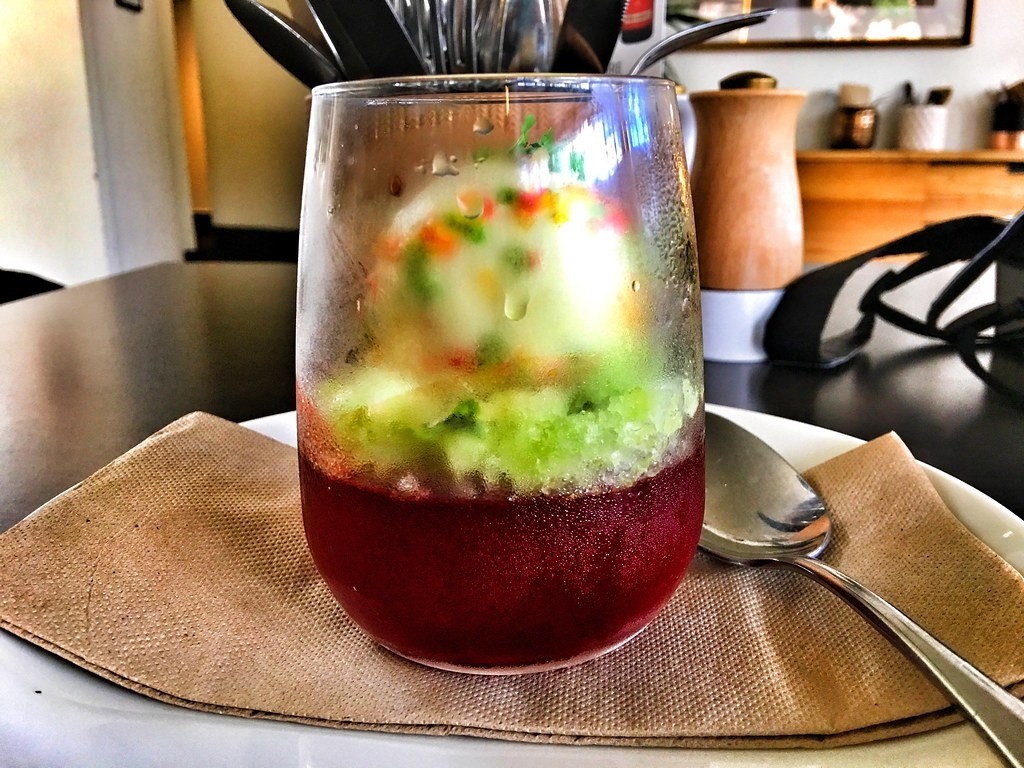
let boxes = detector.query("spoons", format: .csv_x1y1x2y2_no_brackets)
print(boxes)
694,405,1024,768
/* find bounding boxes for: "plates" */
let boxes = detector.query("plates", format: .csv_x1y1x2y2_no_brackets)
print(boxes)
0,399,1024,768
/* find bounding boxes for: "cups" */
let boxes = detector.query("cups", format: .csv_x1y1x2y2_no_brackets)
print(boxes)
292,72,707,673
897,107,948,151
829,105,877,148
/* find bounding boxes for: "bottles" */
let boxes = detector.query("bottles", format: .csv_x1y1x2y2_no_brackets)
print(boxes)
691,88,807,292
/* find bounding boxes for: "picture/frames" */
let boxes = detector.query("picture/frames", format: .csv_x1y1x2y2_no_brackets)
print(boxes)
682,0,976,51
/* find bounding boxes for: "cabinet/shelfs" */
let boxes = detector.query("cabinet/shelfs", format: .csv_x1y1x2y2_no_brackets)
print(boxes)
795,150,1024,263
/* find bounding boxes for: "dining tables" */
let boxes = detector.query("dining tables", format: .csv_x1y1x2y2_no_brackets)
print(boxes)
0,260,1024,534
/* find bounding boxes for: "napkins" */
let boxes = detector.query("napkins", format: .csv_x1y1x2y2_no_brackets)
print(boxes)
0,411,1024,750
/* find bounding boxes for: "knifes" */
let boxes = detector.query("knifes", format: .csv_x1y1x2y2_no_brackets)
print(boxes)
220,0,633,89
